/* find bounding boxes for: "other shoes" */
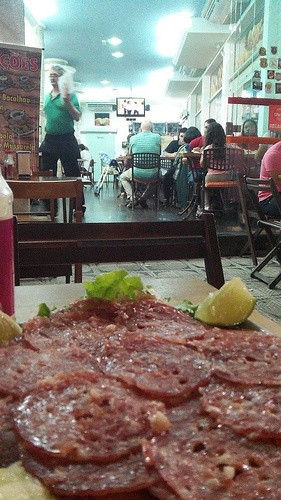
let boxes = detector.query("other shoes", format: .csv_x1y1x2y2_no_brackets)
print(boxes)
127,199,136,208
74,211,84,218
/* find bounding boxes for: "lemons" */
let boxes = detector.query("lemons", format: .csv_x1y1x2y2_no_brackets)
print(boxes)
194,277,256,326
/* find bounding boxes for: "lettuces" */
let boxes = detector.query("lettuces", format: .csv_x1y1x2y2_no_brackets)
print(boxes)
37,269,200,320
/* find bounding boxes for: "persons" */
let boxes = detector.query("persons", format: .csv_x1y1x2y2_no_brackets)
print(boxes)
259,137,281,219
113,117,274,217
37,59,84,220
79,144,92,179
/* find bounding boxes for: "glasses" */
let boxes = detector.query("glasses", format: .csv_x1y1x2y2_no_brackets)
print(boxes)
49,73,58,77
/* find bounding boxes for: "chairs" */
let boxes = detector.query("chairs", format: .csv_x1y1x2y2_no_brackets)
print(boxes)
93,154,281,288
60,157,97,191
4,179,83,288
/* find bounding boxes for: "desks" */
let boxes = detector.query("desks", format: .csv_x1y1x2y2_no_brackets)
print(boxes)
116,154,178,200
0,277,281,500
177,151,256,220
